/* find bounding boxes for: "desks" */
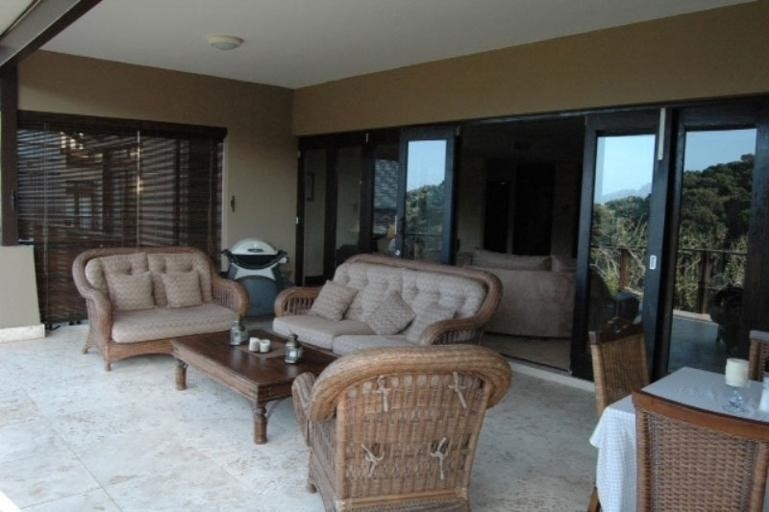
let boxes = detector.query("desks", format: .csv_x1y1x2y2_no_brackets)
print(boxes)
590,361,769,512
169,322,337,446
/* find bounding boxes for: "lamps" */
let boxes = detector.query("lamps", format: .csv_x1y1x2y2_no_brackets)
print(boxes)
208,34,243,50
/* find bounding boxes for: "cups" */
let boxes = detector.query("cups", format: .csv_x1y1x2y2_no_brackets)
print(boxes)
248,337,270,353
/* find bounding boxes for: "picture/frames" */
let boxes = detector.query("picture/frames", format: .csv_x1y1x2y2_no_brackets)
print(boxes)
304,170,316,203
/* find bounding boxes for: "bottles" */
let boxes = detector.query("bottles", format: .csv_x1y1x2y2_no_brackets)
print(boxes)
283,334,301,365
228,318,247,347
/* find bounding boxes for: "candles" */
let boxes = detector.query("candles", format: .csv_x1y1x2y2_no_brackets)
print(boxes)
724,357,754,388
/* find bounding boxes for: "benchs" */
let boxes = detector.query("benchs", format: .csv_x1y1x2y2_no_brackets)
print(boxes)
271,251,502,362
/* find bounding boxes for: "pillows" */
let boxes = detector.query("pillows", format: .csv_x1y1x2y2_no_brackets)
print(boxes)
106,269,156,312
161,268,204,309
312,278,359,322
407,301,455,342
365,288,416,337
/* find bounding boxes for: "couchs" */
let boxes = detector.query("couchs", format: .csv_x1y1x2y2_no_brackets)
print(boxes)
454,246,576,340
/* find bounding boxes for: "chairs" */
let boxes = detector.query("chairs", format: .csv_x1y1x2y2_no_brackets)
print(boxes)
625,381,769,512
71,242,249,374
747,328,769,384
584,312,651,512
288,344,513,512
